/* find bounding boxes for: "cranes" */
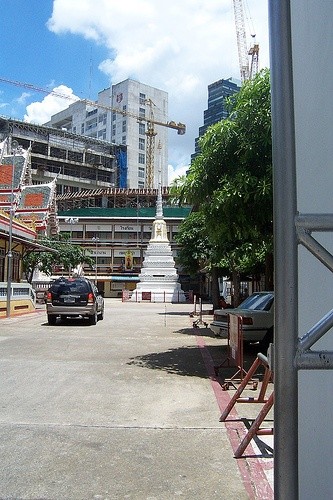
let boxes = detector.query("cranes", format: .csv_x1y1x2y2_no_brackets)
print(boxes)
0,77,186,188
233,0,260,87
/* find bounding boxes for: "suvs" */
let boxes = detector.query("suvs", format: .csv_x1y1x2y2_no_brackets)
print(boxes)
46,277,105,326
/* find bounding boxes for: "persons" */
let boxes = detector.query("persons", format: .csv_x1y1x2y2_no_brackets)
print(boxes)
244,288,249,298
218,296,234,309
121,259,125,272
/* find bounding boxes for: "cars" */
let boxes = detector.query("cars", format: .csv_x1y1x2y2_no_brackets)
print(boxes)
210,291,275,355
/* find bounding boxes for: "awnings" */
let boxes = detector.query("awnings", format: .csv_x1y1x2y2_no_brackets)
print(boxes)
0,232,58,252
